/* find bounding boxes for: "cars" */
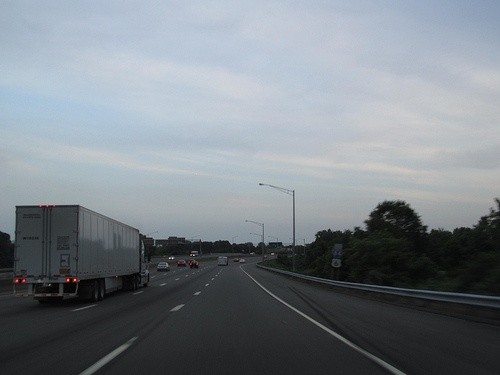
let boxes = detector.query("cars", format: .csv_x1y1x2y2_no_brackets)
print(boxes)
177,260,186,266
189,258,200,268
157,262,170,271
189,251,199,257
232,258,246,263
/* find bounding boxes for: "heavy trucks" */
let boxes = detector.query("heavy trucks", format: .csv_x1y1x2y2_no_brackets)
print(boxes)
13,205,151,300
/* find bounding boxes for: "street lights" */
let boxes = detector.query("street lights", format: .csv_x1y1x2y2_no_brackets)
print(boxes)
259,183,295,273
245,220,264,262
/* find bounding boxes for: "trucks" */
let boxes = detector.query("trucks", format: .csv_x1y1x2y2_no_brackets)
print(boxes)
215,256,229,265
